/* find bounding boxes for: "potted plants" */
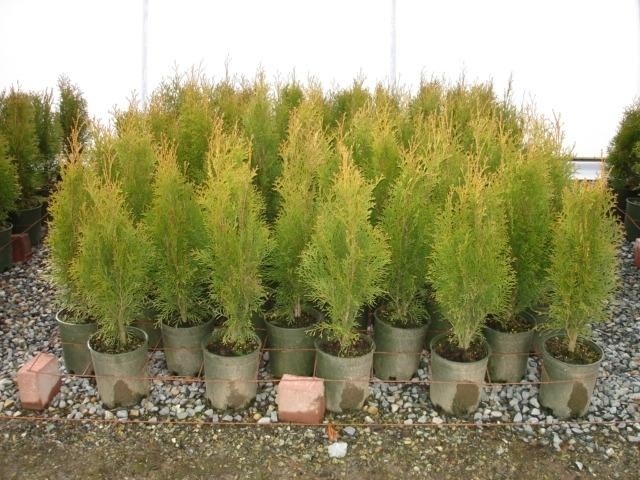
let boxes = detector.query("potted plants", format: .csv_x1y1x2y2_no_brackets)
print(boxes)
538,169,627,419
200,165,271,415
481,175,546,383
252,191,281,347
297,149,389,415
2,88,43,247
42,67,639,222
428,168,517,419
356,304,369,332
525,144,575,358
71,185,154,408
424,291,451,352
372,162,436,382
1,156,20,272
122,148,163,351
263,141,325,377
46,157,100,378
625,197,639,241
147,163,214,377
